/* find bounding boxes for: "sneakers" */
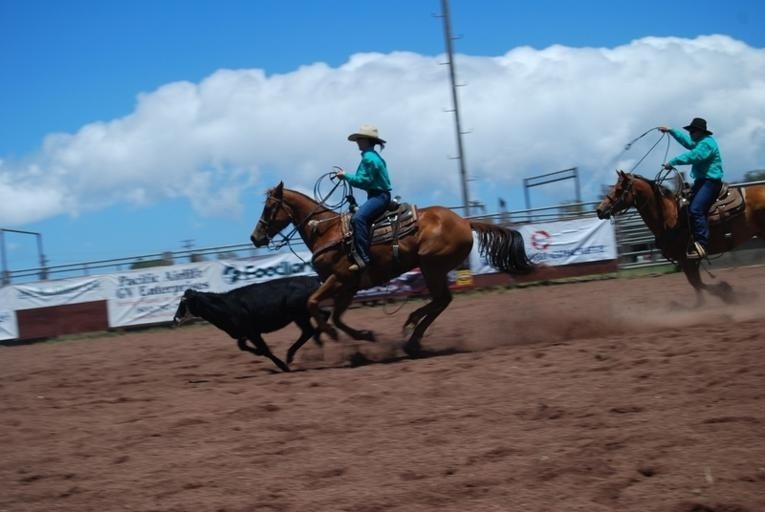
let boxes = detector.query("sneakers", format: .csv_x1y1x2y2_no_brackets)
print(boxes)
687,247,708,259
348,263,359,271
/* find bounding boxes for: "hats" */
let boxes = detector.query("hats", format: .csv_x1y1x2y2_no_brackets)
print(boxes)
347,126,386,145
682,118,713,135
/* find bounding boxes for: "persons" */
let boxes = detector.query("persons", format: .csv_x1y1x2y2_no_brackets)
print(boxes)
659,118,724,259
337,124,392,272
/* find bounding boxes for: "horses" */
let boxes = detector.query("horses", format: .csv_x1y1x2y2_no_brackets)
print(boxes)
596,169,765,308
250,179,539,355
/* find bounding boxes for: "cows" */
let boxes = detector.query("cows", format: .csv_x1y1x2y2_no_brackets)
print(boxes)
174,275,332,372
